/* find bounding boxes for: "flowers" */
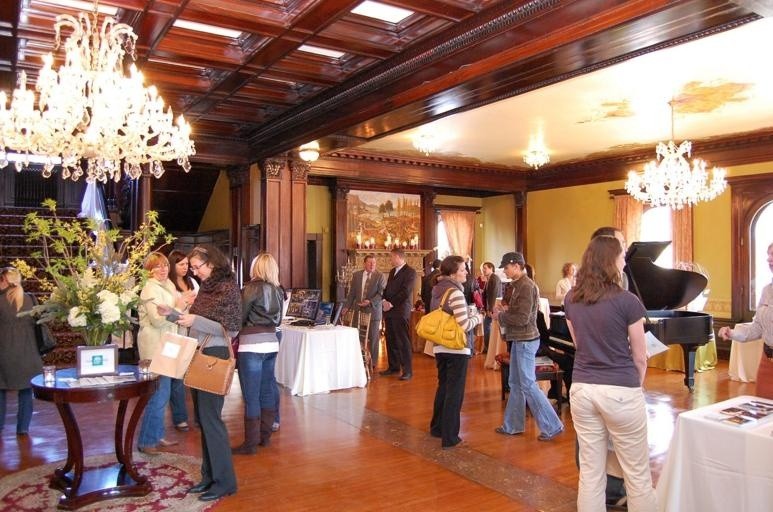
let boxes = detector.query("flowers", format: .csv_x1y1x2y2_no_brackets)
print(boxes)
9,197,179,345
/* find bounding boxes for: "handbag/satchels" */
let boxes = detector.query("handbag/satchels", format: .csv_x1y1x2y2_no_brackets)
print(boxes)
30,291,56,355
413,287,468,349
183,326,237,396
148,331,199,381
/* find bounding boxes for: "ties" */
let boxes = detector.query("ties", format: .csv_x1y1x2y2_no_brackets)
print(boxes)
362,272,371,302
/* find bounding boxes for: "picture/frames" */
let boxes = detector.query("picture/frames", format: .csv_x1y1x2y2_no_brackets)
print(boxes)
76,344,119,378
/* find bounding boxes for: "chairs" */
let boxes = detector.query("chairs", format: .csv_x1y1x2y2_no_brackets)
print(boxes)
340,310,355,327
357,311,373,382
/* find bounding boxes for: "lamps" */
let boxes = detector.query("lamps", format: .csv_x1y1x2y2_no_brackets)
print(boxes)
519,148,551,170
298,149,319,162
624,99,728,211
0,0,198,184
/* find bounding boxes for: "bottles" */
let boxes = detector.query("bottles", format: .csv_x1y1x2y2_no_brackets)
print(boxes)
339,264,359,282
413,292,425,311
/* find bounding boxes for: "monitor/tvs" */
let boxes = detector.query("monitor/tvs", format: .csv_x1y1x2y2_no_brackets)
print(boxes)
285,288,322,326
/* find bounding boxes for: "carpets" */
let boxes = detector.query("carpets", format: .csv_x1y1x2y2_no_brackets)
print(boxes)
0,450,227,512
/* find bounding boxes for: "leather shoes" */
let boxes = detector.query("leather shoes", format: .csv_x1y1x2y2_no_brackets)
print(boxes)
186,482,212,494
159,439,181,445
378,368,414,380
138,445,170,456
175,422,191,432
198,485,236,502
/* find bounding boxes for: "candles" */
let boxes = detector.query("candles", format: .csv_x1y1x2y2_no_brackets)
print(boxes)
356,233,419,250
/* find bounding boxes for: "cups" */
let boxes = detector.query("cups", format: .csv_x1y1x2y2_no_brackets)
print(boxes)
41,366,56,381
139,358,151,374
356,232,419,250
468,305,477,318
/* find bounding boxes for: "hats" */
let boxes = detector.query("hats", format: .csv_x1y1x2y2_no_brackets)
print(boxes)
497,251,526,270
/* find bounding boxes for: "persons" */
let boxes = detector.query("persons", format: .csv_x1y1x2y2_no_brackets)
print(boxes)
381,249,417,380
135,246,284,502
422,258,503,359
489,251,565,440
0,266,41,435
556,263,574,306
575,228,644,509
564,236,654,511
428,255,483,447
718,243,773,400
341,254,386,370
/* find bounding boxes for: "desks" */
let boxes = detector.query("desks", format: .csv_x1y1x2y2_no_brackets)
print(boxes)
30,364,160,511
727,322,764,383
652,394,773,512
273,324,367,397
410,308,426,353
647,328,717,373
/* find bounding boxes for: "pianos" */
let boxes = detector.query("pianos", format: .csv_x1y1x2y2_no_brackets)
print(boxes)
549,242,713,417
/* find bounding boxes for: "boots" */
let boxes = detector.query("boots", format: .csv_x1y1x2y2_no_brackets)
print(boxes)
230,415,261,456
258,407,276,448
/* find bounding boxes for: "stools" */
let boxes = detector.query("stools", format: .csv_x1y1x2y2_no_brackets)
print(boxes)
494,352,565,416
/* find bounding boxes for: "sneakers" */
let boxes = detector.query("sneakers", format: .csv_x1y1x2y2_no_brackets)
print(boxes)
605,495,630,510
442,439,475,449
537,424,566,441
